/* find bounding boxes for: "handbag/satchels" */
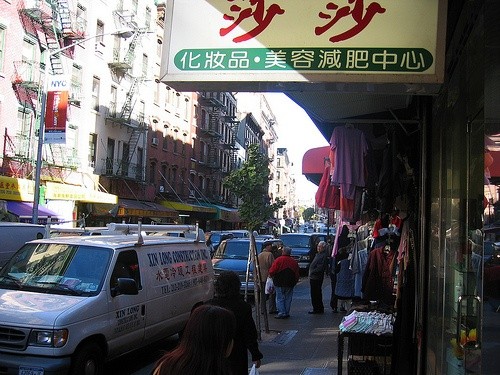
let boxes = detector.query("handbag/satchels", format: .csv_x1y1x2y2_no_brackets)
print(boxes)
264,276,276,294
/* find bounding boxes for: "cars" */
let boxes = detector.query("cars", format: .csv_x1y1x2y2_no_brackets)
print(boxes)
313,220,336,234
204,230,275,249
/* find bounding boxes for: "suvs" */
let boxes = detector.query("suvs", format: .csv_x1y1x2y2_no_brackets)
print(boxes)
279,234,320,269
211,238,283,296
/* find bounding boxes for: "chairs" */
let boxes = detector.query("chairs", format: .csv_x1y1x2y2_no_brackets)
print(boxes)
338,258,406,375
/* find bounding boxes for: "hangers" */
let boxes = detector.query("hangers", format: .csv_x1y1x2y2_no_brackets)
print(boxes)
357,219,406,254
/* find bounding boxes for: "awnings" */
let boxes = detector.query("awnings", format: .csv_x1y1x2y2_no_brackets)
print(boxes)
7,202,64,225
161,201,216,213
195,202,243,222
302,146,331,186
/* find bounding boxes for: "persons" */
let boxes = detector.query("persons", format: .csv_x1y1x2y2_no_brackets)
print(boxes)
151,305,234,375
330,247,349,313
306,234,335,272
258,241,279,314
268,247,299,319
308,241,327,314
205,270,263,375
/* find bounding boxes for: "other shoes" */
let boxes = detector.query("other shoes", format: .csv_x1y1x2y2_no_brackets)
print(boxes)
308,310,323,314
269,310,279,315
332,307,337,313
274,315,290,319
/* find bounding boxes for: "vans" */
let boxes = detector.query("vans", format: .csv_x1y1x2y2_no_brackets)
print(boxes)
0,218,215,375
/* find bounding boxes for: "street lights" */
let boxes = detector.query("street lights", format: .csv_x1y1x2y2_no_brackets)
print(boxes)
32,30,132,225
